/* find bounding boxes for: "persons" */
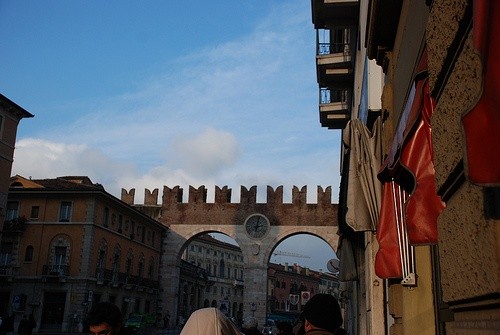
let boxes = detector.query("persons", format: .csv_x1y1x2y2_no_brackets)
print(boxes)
88,294,348,335
17,314,36,335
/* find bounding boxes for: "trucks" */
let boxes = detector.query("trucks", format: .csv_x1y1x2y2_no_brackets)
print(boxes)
124,311,156,333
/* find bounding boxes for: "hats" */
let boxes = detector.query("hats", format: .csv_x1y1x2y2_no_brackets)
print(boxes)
305,292,346,328
